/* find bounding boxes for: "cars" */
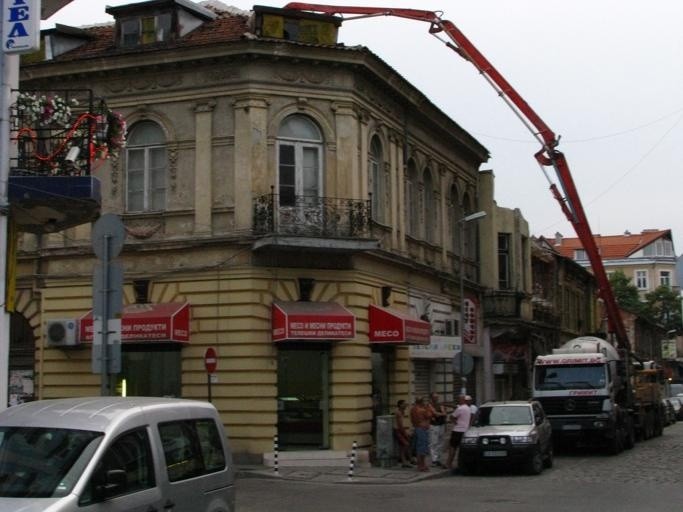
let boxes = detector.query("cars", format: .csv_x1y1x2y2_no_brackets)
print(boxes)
460,400,553,475
662,384,683,424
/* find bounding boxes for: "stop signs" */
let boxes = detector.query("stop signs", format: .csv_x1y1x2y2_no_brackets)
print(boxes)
205,348,217,373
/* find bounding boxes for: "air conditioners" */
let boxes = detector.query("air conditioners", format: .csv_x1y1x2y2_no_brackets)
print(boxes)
45,319,79,347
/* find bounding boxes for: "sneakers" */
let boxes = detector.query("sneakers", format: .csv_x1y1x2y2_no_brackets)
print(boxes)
402,460,447,473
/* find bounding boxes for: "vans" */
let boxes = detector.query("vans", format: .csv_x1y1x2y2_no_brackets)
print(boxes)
0,396,236,512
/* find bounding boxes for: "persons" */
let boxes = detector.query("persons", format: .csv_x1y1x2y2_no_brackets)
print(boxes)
436,394,470,470
438,394,477,428
410,394,433,472
427,389,447,464
392,398,417,469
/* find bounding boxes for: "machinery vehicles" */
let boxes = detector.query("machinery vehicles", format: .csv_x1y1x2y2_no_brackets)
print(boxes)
284,2,666,454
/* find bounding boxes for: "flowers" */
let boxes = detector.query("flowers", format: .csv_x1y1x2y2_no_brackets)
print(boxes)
9,92,79,131
93,110,129,160
